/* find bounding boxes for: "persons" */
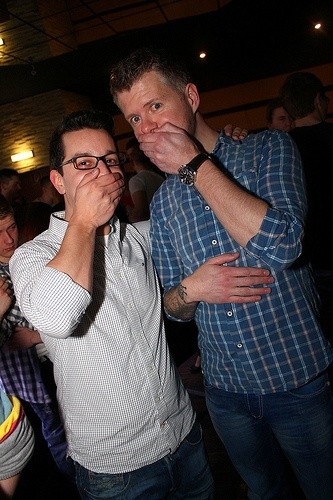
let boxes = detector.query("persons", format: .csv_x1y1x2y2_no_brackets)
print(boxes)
109,47,333,500
9,110,250,500
0,72,333,500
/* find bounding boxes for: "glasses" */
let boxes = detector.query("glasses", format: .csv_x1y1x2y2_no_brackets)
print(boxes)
58,152,127,170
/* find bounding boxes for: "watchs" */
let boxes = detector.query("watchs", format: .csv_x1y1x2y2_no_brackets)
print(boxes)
177,153,212,187
